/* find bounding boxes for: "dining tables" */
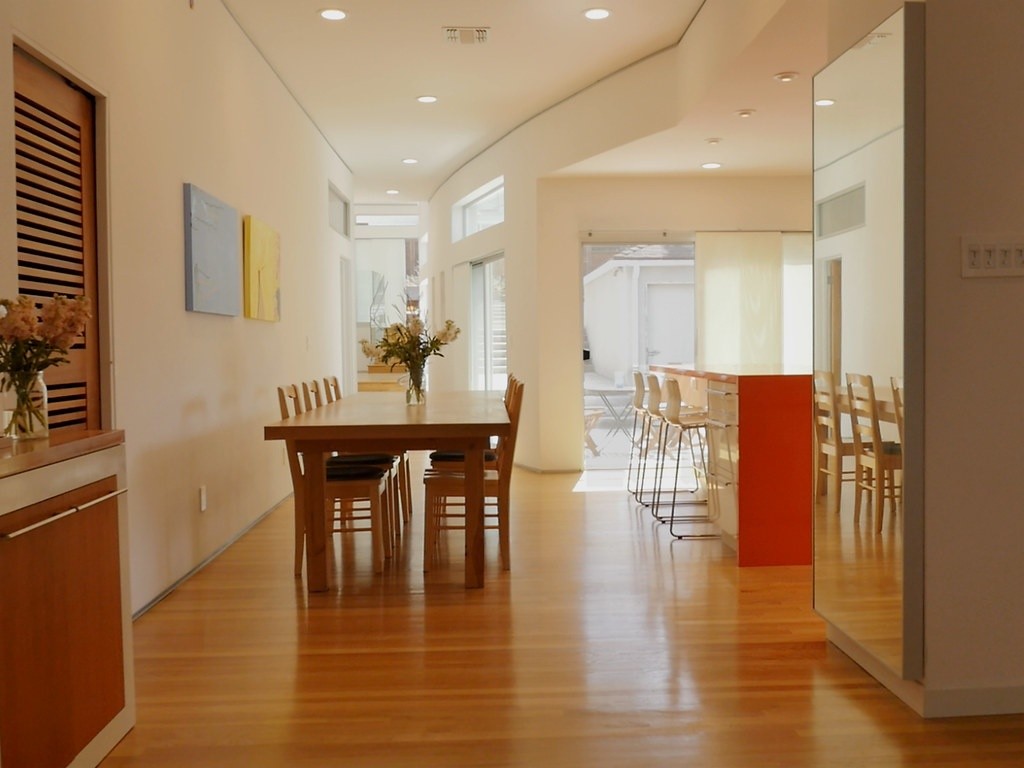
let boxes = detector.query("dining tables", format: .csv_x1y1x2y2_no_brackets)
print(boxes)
264,390,511,592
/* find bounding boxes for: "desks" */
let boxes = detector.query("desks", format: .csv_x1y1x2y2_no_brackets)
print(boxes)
583,385,652,456
650,362,812,567
819,385,905,424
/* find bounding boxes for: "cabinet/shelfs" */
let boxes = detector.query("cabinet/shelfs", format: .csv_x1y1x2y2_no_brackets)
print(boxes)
0,425,136,768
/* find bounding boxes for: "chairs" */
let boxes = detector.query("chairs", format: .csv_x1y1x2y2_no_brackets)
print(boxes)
422,373,525,575
583,395,699,456
814,370,905,541
277,375,414,578
627,369,722,540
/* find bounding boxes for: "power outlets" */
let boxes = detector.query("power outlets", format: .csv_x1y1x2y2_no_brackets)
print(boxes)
960,234,1024,277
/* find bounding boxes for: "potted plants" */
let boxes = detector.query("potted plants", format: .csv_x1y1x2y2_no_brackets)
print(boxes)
358,288,462,407
1,296,92,438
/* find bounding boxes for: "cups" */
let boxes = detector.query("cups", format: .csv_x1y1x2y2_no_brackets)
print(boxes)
614,369,624,387
